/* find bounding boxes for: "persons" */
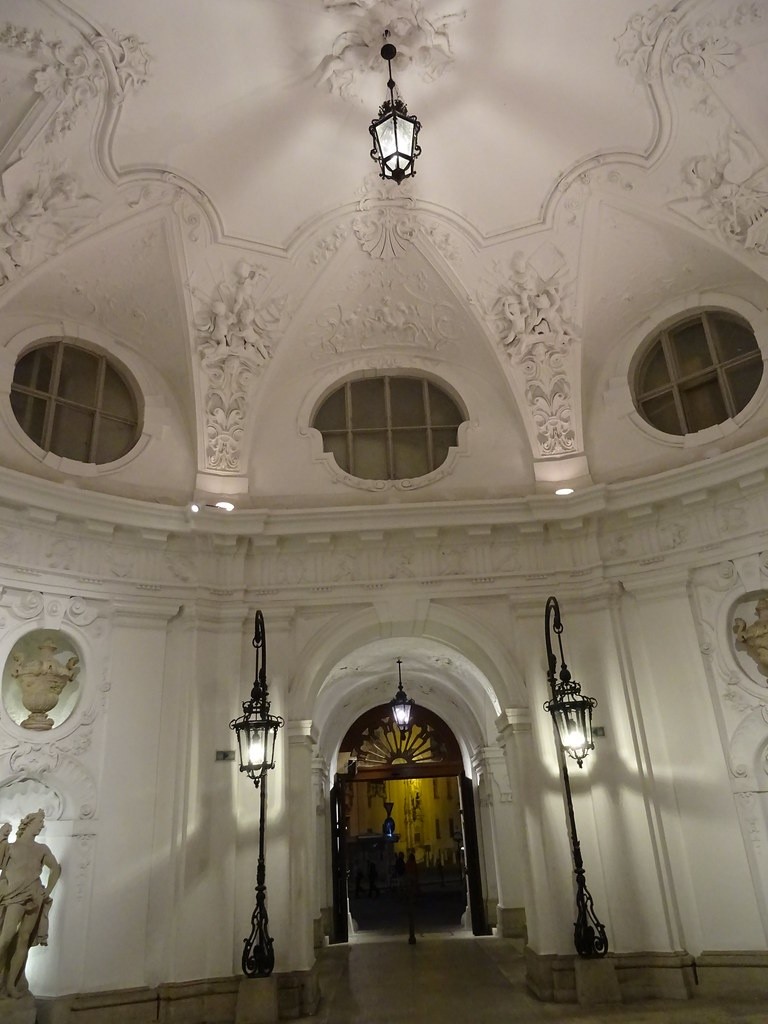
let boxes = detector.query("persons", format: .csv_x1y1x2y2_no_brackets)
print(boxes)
0,807,63,999
211,301,271,359
354,852,421,898
503,288,582,344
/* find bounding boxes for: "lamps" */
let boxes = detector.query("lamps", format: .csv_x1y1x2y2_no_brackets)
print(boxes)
544,595,609,959
369,45,423,185
230,611,285,975
390,659,415,734
